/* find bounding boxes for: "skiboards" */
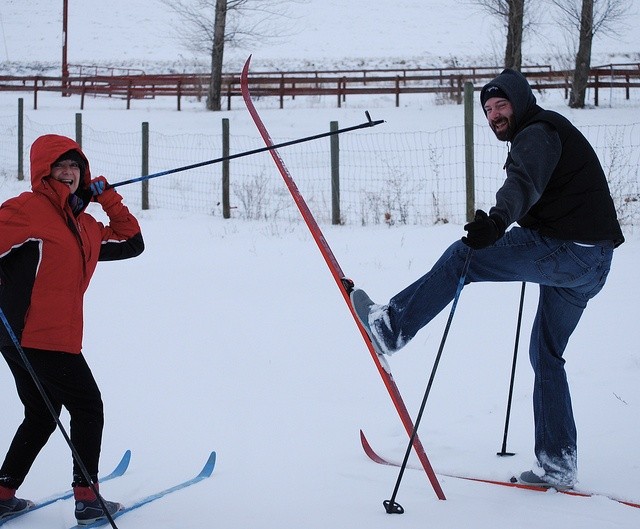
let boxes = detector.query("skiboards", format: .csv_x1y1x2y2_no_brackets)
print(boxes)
241,54,638,507
1,450,217,529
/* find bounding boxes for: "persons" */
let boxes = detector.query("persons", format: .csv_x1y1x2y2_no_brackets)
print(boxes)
0,133,145,525
348,67,626,491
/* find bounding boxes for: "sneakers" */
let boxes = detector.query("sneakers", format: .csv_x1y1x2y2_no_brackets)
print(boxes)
350,288,396,354
519,470,576,489
74,498,120,524
0,497,33,521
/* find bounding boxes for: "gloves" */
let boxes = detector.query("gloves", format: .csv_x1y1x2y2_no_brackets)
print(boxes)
89,177,122,209
461,210,505,248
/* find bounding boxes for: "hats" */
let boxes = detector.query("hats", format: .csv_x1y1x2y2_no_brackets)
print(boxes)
483,85,508,104
